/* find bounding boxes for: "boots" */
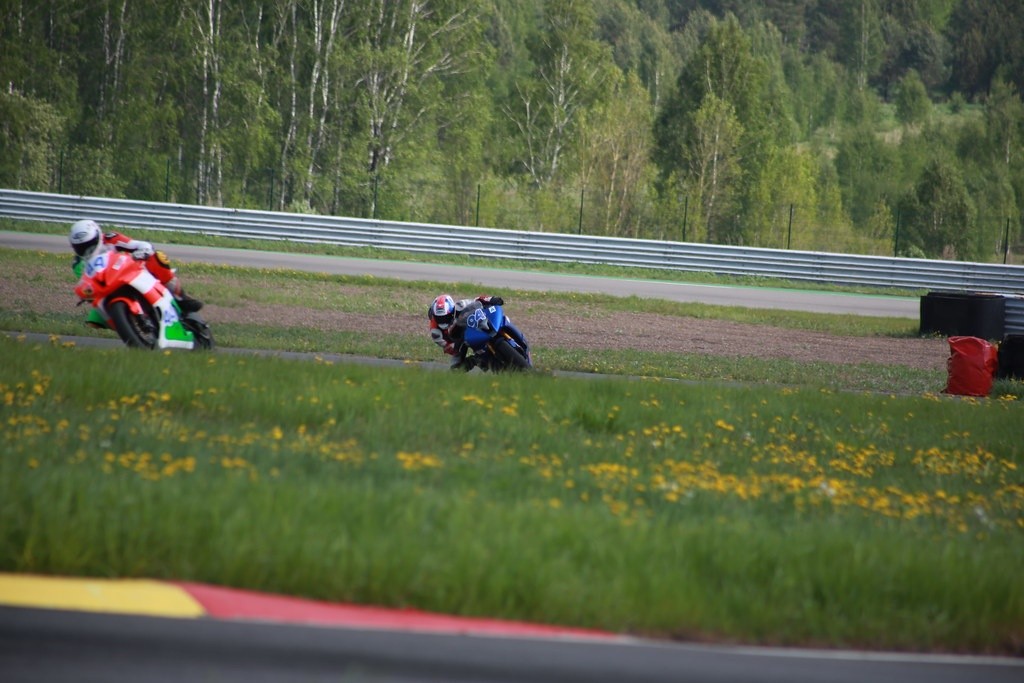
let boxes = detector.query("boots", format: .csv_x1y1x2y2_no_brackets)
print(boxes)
167,276,203,313
465,354,489,373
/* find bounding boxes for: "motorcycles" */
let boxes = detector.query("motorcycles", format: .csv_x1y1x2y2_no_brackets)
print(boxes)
446,300,532,375
72,251,220,350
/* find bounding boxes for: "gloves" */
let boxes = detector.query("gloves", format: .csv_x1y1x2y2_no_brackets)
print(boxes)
132,250,148,260
490,296,503,306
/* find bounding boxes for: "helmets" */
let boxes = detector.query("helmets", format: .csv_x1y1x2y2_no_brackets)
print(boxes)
431,295,456,329
68,220,102,260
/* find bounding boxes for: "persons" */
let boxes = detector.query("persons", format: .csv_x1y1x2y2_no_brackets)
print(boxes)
429,293,505,374
68,218,205,329
